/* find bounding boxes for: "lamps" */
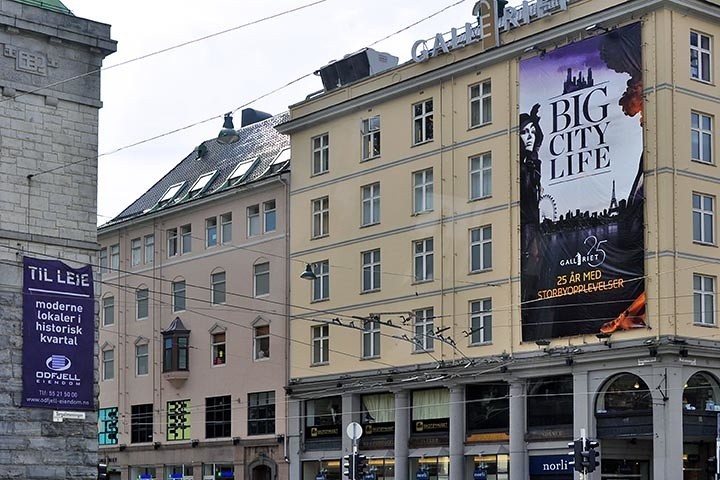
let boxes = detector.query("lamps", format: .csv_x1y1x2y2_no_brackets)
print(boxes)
191,439,199,447
277,434,284,443
585,24,611,33
299,263,317,280
679,346,688,357
565,356,573,367
216,114,241,145
337,381,342,387
523,46,546,57
535,341,552,354
120,444,126,451
233,437,239,444
595,333,612,348
501,366,508,373
285,388,292,394
650,347,657,357
154,442,161,450
386,375,393,382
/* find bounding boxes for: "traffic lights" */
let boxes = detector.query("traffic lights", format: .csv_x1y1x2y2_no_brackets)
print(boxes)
344,453,355,480
355,452,366,480
584,438,599,473
97,461,109,480
568,438,584,474
